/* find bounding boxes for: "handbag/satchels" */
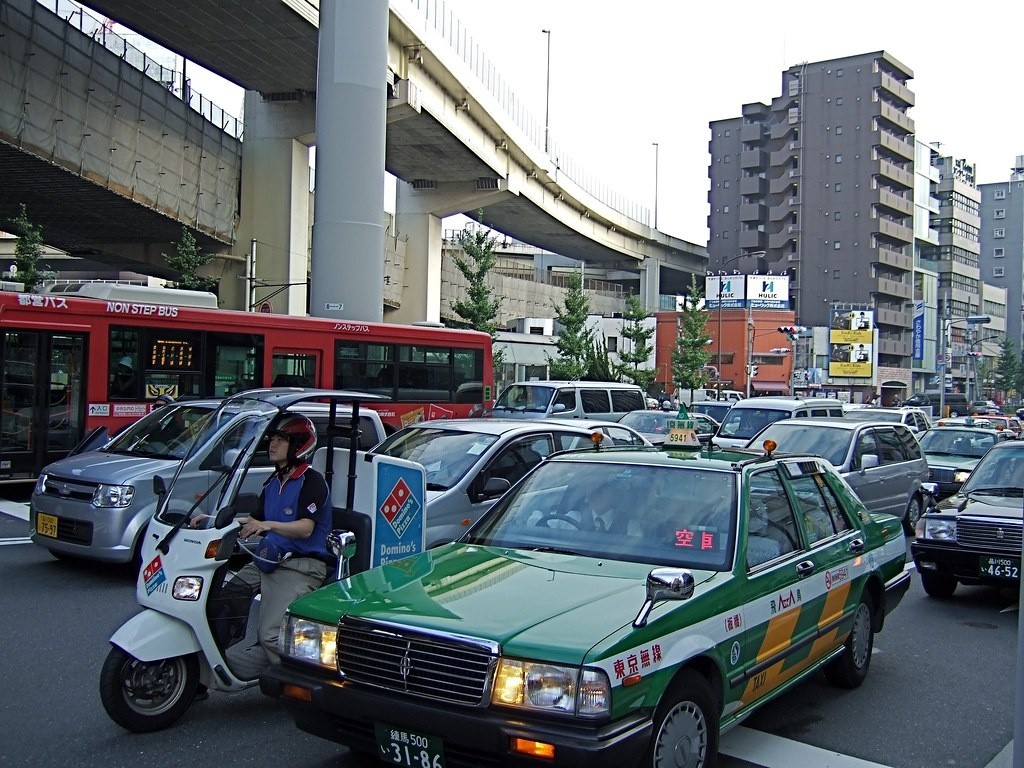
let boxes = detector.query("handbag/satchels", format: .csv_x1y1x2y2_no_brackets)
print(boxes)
254,539,281,573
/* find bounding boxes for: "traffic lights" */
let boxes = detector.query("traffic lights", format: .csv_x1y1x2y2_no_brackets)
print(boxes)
777,325,806,335
977,357,981,362
749,364,758,378
967,351,980,357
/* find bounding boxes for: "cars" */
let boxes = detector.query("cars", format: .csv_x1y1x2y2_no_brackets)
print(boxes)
933,418,991,429
741,417,930,518
259,451,910,768
369,418,650,553
969,400,999,415
30,401,392,583
970,416,1024,440
917,426,1016,492
911,441,1024,601
1016,408,1024,420
617,410,720,452
697,389,745,423
844,405,931,441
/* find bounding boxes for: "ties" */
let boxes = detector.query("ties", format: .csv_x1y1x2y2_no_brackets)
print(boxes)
596,517,606,533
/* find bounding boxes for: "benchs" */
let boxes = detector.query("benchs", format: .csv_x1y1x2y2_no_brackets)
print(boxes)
746,493,831,550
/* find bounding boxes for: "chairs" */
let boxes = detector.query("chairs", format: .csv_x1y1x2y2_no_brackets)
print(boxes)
319,505,373,587
717,495,782,567
489,451,543,488
272,373,379,394
604,485,659,540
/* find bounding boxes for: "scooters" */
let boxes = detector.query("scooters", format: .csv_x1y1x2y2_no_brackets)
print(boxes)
100,386,426,733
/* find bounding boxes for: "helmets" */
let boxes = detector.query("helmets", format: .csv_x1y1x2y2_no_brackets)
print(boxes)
267,410,317,466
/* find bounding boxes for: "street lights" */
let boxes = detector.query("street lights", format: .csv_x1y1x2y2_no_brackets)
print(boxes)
716,251,767,402
971,335,998,402
938,316,990,418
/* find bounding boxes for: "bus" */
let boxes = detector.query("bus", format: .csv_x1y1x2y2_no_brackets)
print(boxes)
0,291,494,485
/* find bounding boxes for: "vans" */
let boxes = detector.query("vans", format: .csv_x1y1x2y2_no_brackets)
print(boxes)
38,282,218,311
703,396,844,472
901,393,969,419
492,378,647,424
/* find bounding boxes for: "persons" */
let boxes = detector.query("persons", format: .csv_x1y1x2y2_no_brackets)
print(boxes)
673,396,683,411
190,411,332,702
831,312,849,330
856,312,868,330
855,344,868,364
830,344,847,362
540,472,645,538
151,393,176,411
